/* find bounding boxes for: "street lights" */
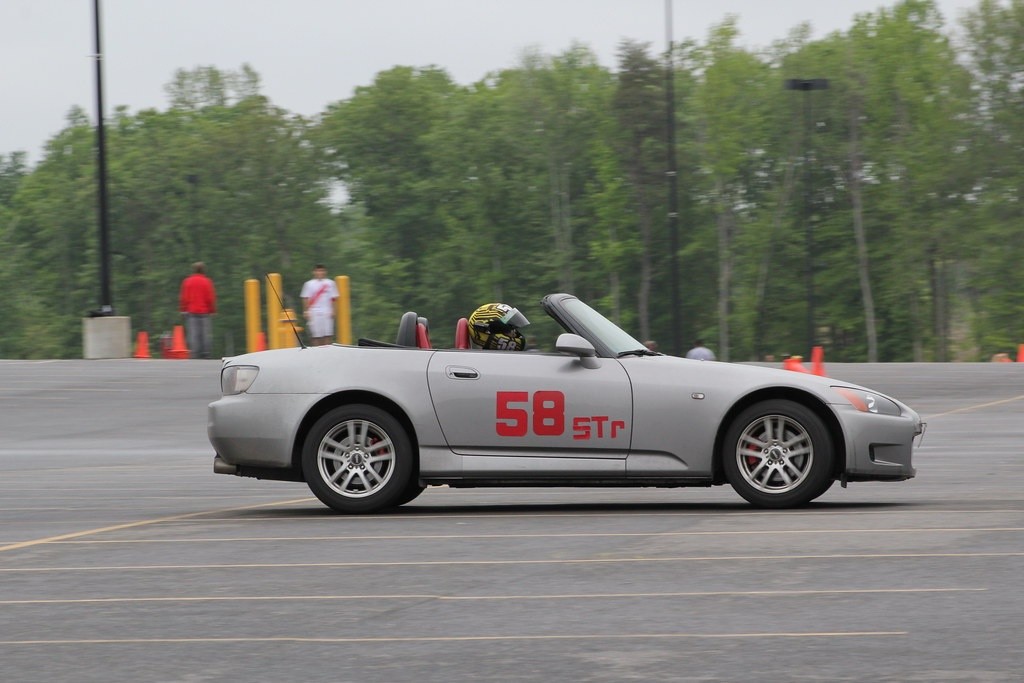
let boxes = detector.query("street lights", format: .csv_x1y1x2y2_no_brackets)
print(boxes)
781,77,832,362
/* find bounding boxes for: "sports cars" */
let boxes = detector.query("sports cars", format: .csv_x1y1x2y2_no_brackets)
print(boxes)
203,292,927,514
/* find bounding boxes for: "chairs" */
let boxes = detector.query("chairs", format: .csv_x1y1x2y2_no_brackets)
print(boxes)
455,318,473,350
395,311,434,349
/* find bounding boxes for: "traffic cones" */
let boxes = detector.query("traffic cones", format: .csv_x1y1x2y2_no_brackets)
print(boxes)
810,345,828,377
991,353,1013,362
130,330,152,359
1015,342,1024,363
166,325,191,358
257,332,267,351
784,355,810,374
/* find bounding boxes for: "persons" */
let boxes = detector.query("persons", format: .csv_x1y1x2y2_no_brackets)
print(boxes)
179,262,216,359
300,263,339,346
467,303,530,351
685,338,717,361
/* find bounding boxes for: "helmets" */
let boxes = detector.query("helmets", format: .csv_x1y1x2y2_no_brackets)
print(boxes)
468,303,525,352
